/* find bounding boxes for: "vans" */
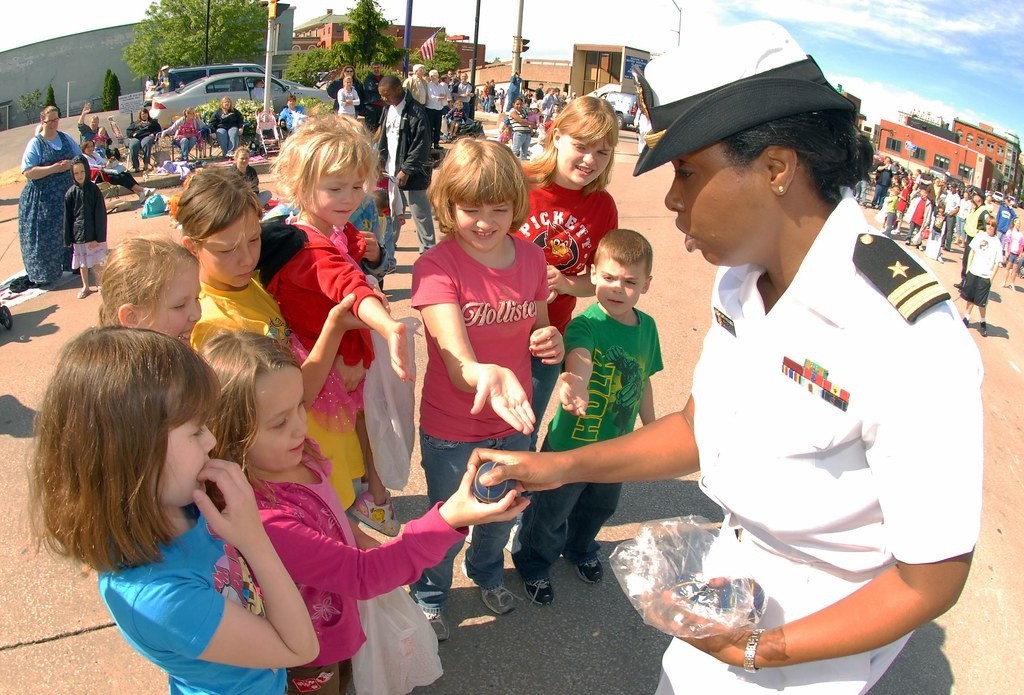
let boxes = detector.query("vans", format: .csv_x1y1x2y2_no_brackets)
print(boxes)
929,168,964,196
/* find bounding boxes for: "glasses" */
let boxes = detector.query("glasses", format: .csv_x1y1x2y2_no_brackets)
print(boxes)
986,222,996,228
44,118,60,124
344,67,380,73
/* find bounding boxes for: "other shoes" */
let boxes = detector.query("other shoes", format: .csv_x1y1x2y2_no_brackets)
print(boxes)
77,286,101,299
939,240,966,264
872,205,881,210
953,281,963,293
879,227,901,238
906,240,923,251
139,188,155,205
385,266,396,274
1001,283,1017,292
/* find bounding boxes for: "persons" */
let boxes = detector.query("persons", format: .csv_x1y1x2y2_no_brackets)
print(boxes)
99,234,203,350
468,21,984,695
198,332,530,695
175,167,445,695
27,326,320,695
19,60,1024,622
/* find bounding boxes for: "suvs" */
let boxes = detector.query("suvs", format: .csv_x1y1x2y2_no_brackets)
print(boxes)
595,91,640,130
156,65,304,94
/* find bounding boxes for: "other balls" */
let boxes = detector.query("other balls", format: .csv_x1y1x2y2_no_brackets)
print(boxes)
470,463,516,505
673,574,764,621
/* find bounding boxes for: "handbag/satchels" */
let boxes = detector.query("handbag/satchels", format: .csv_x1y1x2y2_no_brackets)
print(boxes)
913,230,943,260
142,194,167,218
633,113,641,133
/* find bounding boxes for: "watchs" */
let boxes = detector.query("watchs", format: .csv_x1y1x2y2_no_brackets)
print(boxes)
743,629,765,673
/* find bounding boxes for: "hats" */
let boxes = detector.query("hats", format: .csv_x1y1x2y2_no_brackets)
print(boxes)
412,64,425,74
160,65,169,71
635,19,855,173
970,188,986,200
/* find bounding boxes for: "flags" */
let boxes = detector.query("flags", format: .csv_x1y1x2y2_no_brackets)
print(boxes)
419,32,437,61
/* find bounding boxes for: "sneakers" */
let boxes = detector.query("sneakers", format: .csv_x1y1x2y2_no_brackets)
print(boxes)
420,604,449,640
462,555,520,613
963,318,987,336
574,557,603,583
524,578,554,606
348,489,401,538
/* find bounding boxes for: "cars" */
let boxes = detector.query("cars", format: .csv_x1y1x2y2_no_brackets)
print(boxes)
309,69,338,92
144,85,161,105
992,190,1024,210
148,72,329,129
965,184,986,201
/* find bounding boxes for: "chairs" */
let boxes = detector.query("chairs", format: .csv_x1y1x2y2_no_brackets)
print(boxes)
89,115,257,200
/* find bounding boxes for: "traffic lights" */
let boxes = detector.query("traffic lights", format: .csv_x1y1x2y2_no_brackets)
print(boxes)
522,39,530,52
